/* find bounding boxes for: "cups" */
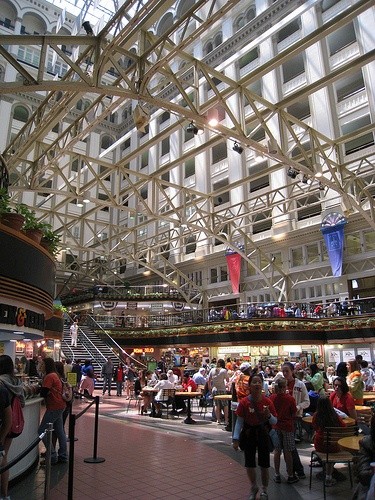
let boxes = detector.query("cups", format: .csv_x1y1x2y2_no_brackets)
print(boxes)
366,385,372,390
187,386,192,392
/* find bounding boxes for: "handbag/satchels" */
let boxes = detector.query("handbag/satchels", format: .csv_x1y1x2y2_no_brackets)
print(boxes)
231,401,239,411
267,429,280,448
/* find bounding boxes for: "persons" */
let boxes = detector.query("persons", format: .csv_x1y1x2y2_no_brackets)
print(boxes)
171,371,197,415
0,354,26,500
114,362,124,396
122,362,136,400
269,377,300,484
150,373,175,418
79,358,96,399
71,359,81,397
41,362,71,456
0,379,12,455
135,370,151,413
282,362,310,437
328,378,358,423
0,297,375,500
69,322,78,347
312,394,345,485
38,358,69,464
233,374,277,500
64,358,72,380
100,356,114,397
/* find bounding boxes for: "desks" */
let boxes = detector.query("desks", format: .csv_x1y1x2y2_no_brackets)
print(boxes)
302,416,355,426
338,434,370,462
143,389,160,417
214,394,232,431
175,391,203,424
355,405,371,413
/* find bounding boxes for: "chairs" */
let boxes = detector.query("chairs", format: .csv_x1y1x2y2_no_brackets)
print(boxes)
126,384,375,500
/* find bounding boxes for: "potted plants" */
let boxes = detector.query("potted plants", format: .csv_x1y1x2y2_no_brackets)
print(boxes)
0,186,61,256
53,304,67,317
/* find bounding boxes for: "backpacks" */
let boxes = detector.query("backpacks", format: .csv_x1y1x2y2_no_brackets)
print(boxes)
1,380,25,436
52,377,74,401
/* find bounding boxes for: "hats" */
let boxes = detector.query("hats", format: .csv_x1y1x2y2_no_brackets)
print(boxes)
239,362,252,372
156,367,162,372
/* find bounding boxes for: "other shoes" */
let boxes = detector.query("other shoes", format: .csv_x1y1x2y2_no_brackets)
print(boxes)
288,476,298,482
40,459,49,465
149,412,156,417
273,474,280,482
326,478,336,486
249,488,259,500
295,468,305,476
156,412,162,417
41,451,47,457
259,489,268,497
179,408,188,414
309,458,325,467
233,439,238,449
126,397,129,400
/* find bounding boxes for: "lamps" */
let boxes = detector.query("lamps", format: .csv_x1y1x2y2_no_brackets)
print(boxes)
287,167,296,178
186,123,198,135
233,141,243,153
302,176,308,183
